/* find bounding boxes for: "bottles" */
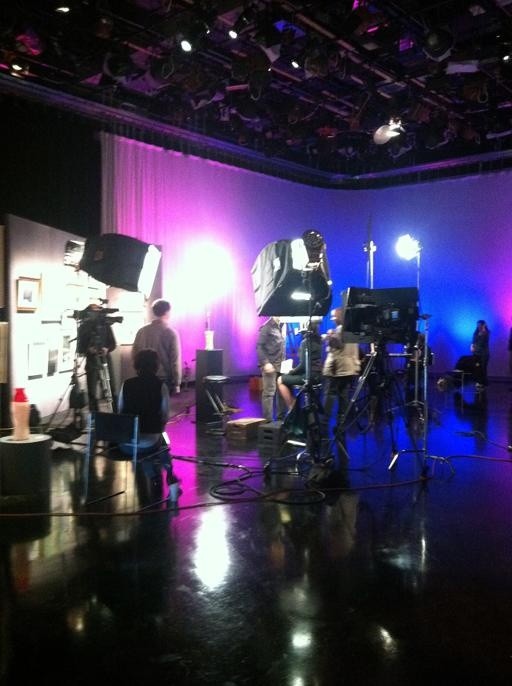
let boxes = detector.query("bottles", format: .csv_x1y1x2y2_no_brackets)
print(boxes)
10,388,31,441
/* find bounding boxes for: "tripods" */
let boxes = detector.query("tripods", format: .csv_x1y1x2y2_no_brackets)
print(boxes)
46,353,118,431
264,319,349,479
325,354,433,495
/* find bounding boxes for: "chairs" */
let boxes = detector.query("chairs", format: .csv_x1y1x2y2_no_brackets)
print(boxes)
86,411,171,520
446,355,478,410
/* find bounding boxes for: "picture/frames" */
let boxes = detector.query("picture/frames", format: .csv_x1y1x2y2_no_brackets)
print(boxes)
17,274,44,313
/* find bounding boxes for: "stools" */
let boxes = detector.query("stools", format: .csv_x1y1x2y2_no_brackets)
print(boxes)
291,383,323,430
201,375,239,437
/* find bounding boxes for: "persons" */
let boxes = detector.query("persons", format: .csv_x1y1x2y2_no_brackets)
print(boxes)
409,332,433,385
277,320,323,409
470,320,491,392
115,349,181,485
76,299,119,414
255,316,287,422
130,298,182,397
321,307,362,439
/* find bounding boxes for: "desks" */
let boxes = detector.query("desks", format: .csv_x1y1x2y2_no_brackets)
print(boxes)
0,432,55,544
364,352,415,421
